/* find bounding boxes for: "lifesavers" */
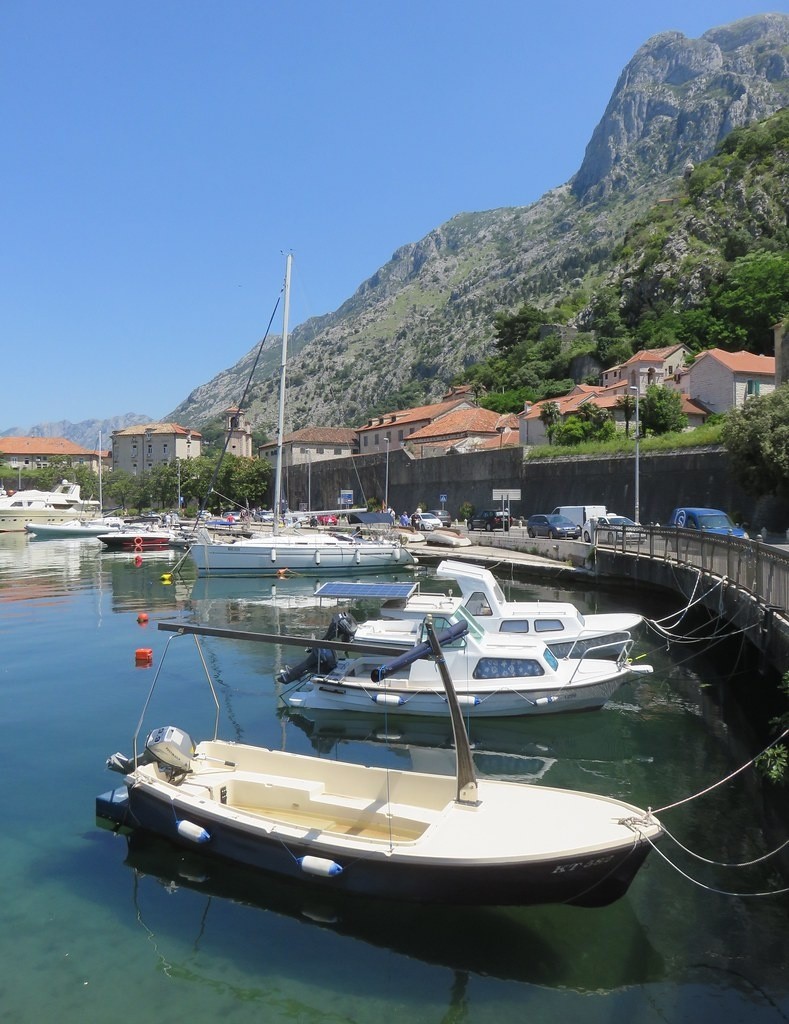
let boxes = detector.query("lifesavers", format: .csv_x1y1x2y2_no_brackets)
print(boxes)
134,545,143,552
134,537,143,545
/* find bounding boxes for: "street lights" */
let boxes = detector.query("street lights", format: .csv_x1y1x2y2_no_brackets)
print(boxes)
383,437,390,508
629,385,640,523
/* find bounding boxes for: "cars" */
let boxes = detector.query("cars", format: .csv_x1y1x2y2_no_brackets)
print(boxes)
527,513,581,539
581,515,647,545
196,509,275,522
318,514,338,525
407,512,444,531
667,507,750,555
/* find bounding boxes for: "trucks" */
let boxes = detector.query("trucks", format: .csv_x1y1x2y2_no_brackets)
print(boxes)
551,505,606,528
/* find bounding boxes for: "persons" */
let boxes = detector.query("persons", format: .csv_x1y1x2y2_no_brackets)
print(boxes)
387,506,422,527
227,506,261,529
353,526,362,538
165,512,171,528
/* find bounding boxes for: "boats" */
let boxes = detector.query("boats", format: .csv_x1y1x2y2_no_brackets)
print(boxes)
0,478,101,532
275,559,652,725
93,629,668,909
95,524,254,551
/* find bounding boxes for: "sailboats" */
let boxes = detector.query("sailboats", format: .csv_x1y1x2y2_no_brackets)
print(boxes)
24,430,122,536
186,247,422,579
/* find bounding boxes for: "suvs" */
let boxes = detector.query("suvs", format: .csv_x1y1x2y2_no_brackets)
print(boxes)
466,508,513,532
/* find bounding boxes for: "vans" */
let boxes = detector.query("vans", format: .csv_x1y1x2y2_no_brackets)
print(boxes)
428,509,451,527
